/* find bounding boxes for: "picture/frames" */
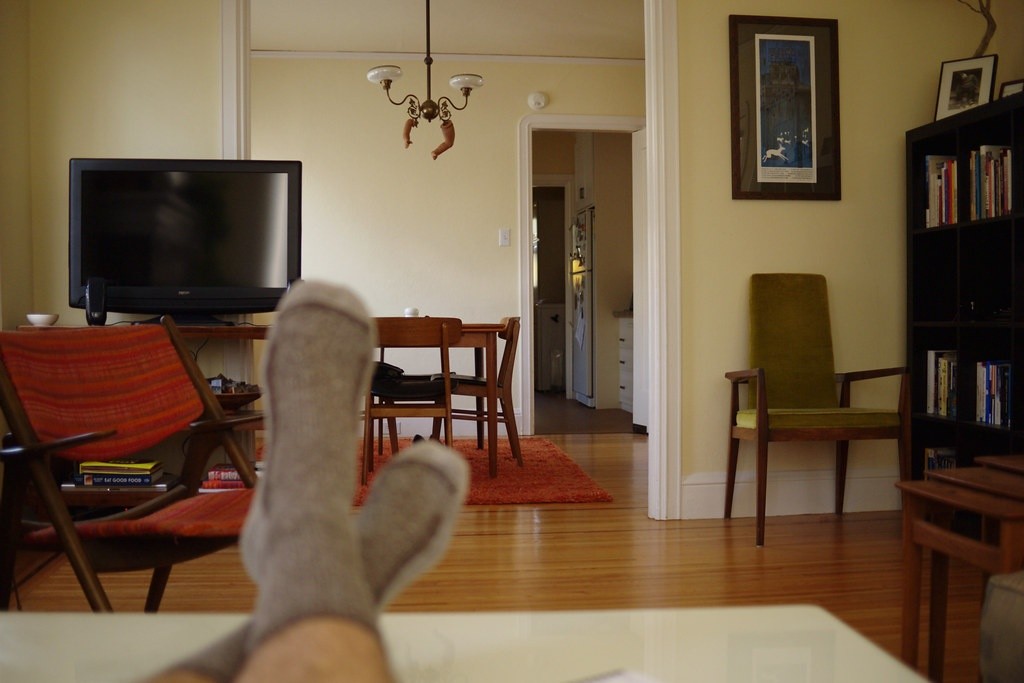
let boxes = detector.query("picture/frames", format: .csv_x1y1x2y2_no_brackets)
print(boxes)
934,54,998,122
729,14,842,201
999,79,1024,99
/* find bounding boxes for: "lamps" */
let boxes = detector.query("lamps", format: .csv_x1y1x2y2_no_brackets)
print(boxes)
366,0,485,159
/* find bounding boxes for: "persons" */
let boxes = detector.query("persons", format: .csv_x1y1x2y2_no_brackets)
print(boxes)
957,70,978,104
130,276,471,683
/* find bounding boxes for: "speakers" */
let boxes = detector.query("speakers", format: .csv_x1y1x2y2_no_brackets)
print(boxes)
85,278,107,327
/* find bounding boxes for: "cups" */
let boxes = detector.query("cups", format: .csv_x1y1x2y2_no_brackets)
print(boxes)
405,308,419,317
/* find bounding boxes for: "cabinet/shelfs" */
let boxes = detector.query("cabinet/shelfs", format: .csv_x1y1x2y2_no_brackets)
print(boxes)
612,310,633,413
19,325,271,522
534,301,566,391
906,91,1024,537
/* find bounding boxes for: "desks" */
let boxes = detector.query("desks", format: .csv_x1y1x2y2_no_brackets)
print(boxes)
0,603,930,683
354,322,506,478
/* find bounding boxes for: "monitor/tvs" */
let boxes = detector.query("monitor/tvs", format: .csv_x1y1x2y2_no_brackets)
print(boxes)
69,158,303,326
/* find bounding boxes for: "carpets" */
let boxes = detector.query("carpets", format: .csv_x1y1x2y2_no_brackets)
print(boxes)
352,436,612,507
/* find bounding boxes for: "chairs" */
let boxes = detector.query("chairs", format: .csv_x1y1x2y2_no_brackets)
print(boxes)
724,273,913,547
360,318,462,486
894,454,1024,683
0,314,270,611
431,316,523,467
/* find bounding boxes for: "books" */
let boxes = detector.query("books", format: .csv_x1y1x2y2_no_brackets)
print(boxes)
198,460,262,493
925,143,1013,229
926,350,1012,427
59,458,178,492
924,447,960,482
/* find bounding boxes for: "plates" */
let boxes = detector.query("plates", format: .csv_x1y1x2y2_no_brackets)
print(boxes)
26,314,59,326
